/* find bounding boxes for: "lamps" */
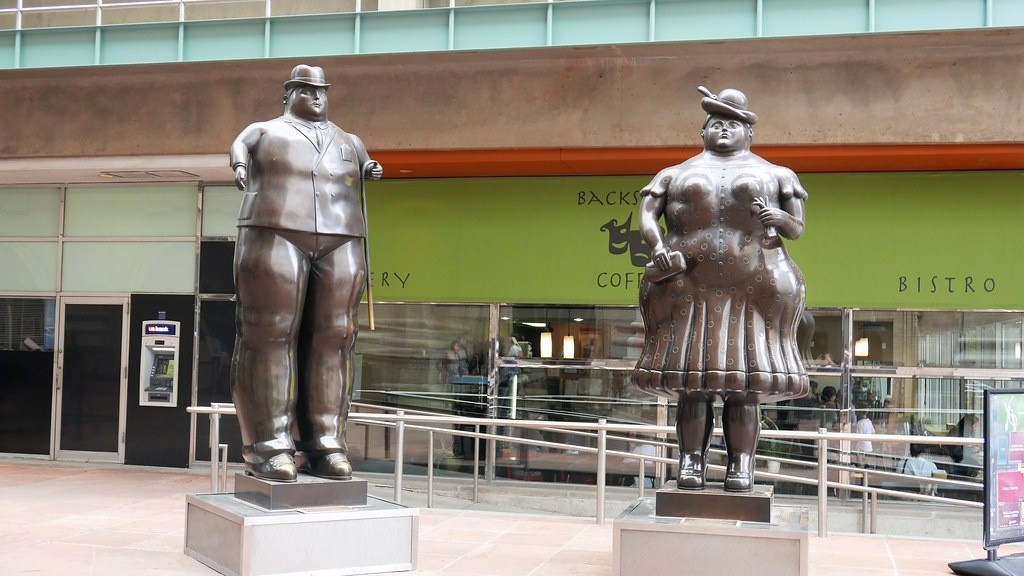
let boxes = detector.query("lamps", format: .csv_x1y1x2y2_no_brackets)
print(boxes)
563,309,574,359
541,309,552,358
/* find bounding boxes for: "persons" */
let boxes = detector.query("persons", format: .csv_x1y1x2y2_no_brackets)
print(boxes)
631,380,980,497
632,86,813,493
229,63,382,484
